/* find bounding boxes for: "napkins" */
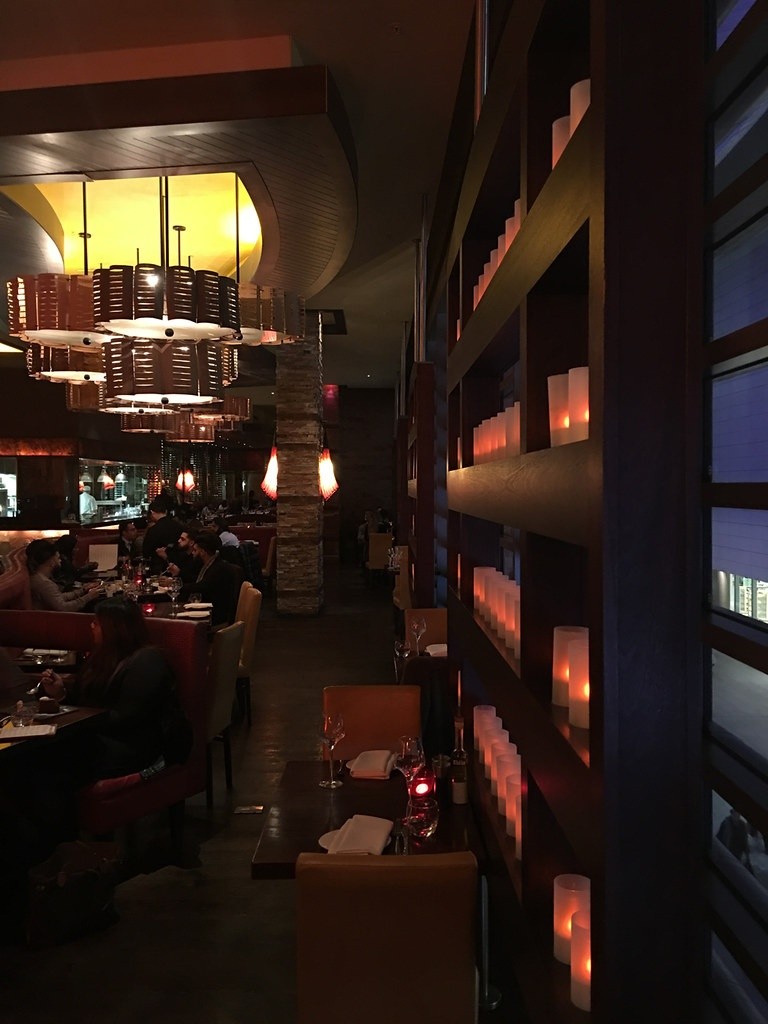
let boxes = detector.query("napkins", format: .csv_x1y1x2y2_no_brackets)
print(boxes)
350,750,395,780
327,815,394,856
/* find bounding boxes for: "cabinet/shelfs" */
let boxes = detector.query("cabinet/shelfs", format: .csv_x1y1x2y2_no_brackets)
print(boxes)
391,362,435,613
449,0,716,1024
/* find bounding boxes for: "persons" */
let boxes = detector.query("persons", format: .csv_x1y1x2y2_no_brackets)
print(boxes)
52,535,98,590
156,488,197,523
357,505,390,542
27,596,180,883
117,519,145,567
202,504,213,515
28,539,100,611
192,533,232,625
219,500,229,511
211,517,240,548
143,502,176,576
156,530,198,582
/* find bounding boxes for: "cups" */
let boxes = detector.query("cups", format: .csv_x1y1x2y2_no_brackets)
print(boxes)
547,366,588,447
570,908,591,1011
473,402,520,464
474,567,520,659
432,754,451,800
395,640,410,658
11,711,34,728
406,798,440,837
551,626,590,728
554,874,591,965
474,704,522,862
473,81,591,307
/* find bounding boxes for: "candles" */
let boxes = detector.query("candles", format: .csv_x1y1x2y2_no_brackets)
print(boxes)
550,626,588,731
457,319,460,342
472,706,521,863
552,872,591,1012
473,567,521,662
473,400,521,465
471,201,522,311
457,448,460,468
552,78,590,171
549,366,589,447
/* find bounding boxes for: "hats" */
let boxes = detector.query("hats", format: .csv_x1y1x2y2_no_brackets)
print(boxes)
79,480,84,486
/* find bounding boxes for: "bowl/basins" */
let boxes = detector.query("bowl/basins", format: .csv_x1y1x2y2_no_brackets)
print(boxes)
41,699,59,713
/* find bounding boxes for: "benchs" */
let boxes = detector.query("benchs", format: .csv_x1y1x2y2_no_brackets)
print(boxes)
0,544,34,611
0,609,212,860
68,524,277,574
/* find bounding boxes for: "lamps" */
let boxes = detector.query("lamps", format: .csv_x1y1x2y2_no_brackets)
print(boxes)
183,470,195,492
80,466,94,482
104,479,116,490
175,472,184,492
316,447,339,502
114,466,129,482
97,466,114,483
260,446,278,502
6,171,307,445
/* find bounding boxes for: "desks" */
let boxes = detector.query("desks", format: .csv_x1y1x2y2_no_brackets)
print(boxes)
251,759,487,882
0,706,108,756
96,585,170,602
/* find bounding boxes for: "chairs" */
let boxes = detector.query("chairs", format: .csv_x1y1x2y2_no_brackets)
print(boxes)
211,580,263,792
393,545,413,610
323,685,423,761
295,853,478,1024
369,533,394,570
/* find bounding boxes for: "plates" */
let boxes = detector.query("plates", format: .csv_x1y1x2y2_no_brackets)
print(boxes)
188,615,205,617
189,607,207,609
346,759,356,768
319,830,391,851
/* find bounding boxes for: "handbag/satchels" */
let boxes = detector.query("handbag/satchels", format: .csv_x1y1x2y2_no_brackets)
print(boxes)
27,839,121,943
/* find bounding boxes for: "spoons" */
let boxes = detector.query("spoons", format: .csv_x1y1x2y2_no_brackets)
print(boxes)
26,675,45,694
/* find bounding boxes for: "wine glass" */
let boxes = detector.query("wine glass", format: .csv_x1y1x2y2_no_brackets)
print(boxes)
410,616,426,656
319,712,345,788
387,547,402,568
394,737,423,799
167,576,183,615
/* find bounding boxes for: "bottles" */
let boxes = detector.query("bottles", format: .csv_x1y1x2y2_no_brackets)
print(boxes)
121,559,146,586
451,726,467,780
